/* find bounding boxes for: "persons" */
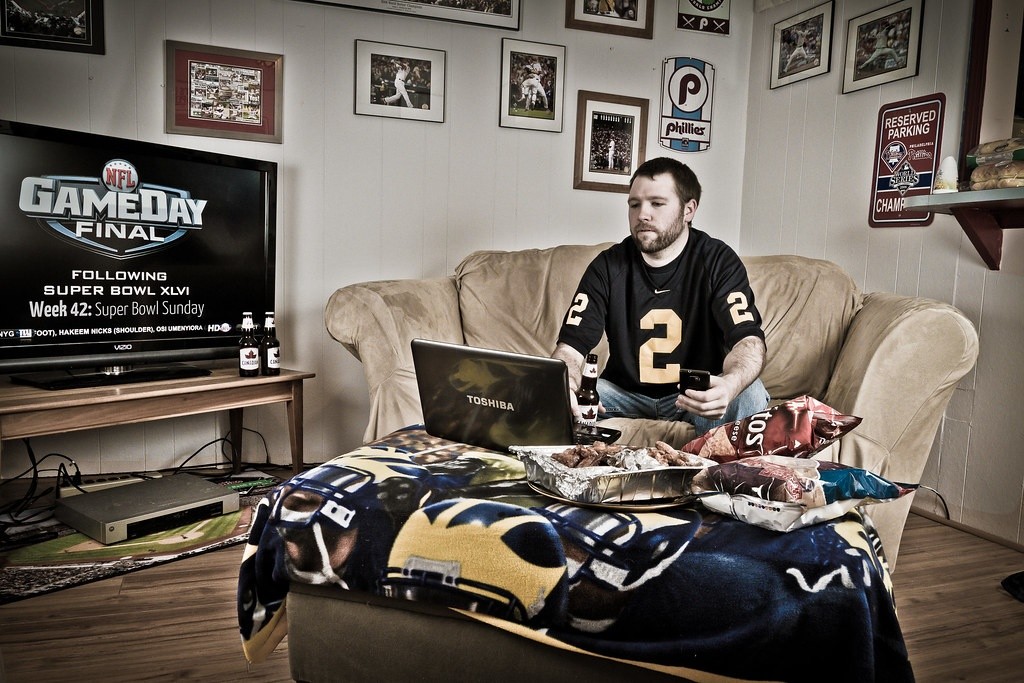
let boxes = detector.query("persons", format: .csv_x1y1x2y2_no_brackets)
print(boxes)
551,157,770,439
371,0,912,177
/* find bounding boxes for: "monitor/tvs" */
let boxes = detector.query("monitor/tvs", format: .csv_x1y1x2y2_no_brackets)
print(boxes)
0,120,279,394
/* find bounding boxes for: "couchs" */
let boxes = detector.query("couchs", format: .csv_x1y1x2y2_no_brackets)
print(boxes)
324,245,977,581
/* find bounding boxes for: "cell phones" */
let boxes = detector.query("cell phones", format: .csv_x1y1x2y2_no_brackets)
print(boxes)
679,369,710,397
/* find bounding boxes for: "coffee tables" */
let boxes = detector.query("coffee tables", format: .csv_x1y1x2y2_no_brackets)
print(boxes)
233,423,919,682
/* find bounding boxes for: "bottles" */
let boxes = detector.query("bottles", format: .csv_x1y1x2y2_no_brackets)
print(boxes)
239,312,260,378
260,312,281,376
575,354,600,426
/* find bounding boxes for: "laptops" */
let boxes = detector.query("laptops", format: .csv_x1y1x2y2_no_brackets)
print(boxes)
410,338,622,456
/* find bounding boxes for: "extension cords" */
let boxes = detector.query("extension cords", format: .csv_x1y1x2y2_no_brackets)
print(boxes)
57,472,162,498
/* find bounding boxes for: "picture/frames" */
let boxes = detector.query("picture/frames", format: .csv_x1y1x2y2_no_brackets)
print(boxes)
769,0,835,89
573,89,649,193
164,39,284,144
354,38,446,124
1,0,104,55
841,0,924,94
565,0,654,39
497,38,565,133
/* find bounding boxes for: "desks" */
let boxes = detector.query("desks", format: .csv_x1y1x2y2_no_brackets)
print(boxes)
0,364,316,478
